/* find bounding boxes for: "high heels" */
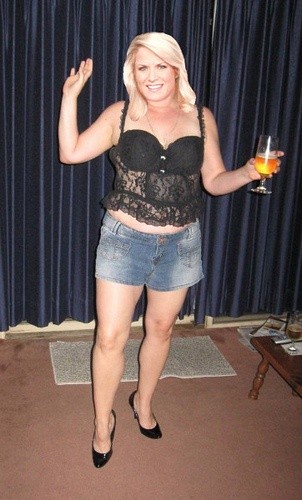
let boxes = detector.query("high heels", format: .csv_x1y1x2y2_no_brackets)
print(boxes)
129,390,162,439
92,409,116,468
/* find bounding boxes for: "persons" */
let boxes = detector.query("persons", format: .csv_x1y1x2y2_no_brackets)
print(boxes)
57,31,287,468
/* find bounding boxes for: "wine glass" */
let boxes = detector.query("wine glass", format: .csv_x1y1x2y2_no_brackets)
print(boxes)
287,311,302,354
250,134,280,194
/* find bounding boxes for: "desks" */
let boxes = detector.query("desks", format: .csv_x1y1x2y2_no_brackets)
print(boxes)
248,336,302,400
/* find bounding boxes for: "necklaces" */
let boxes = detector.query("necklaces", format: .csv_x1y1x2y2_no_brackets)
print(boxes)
144,104,182,149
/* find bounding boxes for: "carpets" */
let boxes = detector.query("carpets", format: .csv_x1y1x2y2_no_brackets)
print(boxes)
49,335,237,385
